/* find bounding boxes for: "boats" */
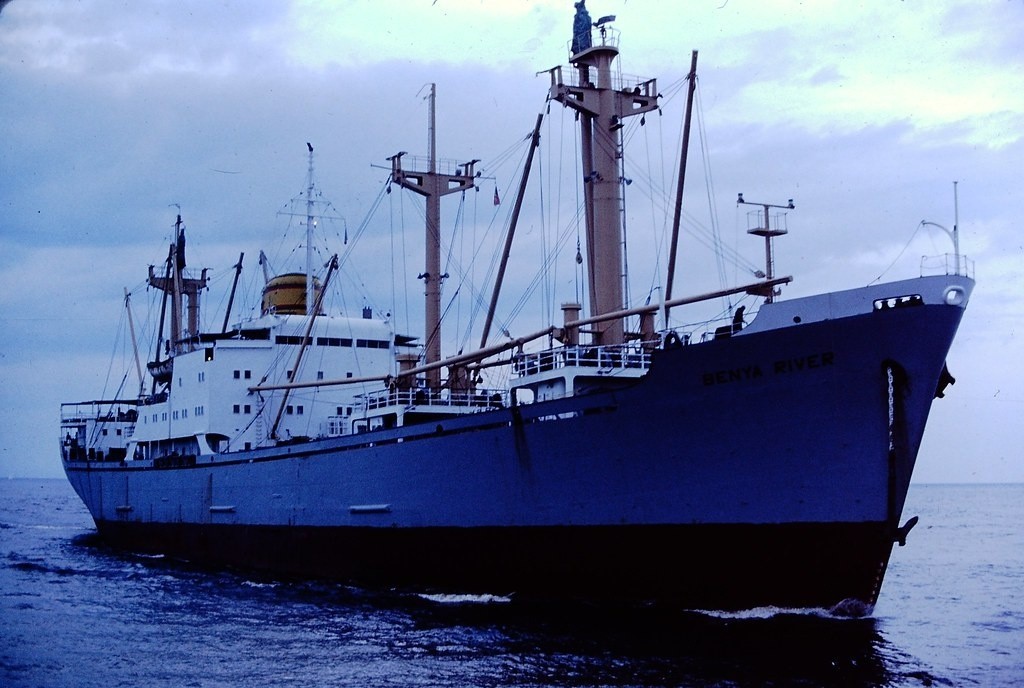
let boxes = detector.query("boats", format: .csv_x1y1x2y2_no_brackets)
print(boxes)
56,0,975,615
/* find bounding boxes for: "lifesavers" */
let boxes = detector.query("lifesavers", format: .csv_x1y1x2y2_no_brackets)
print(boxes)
664,331,679,349
